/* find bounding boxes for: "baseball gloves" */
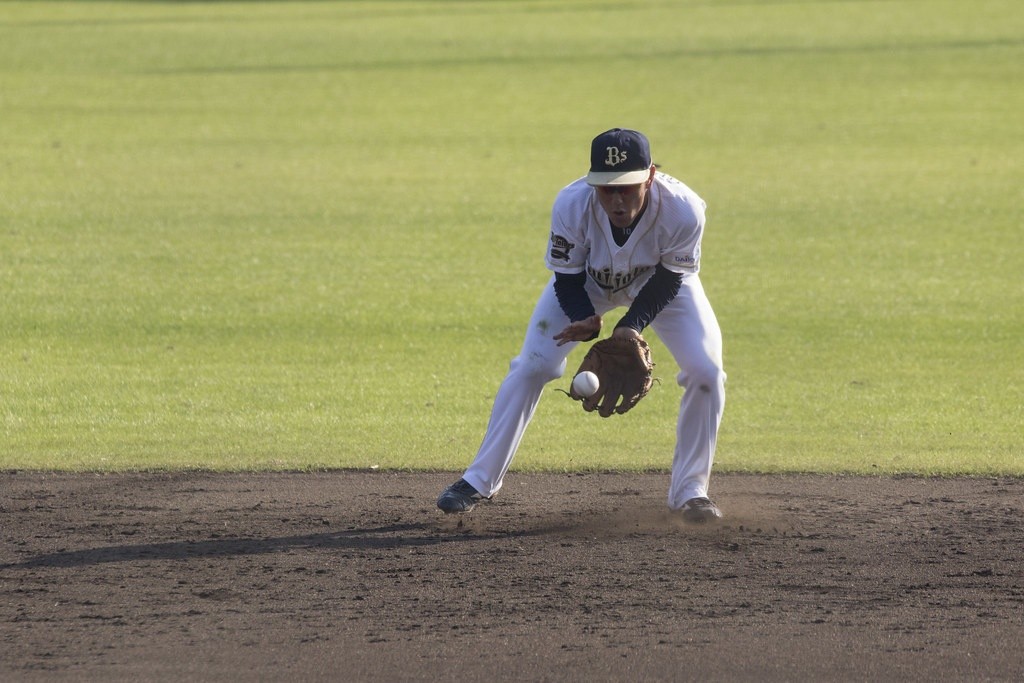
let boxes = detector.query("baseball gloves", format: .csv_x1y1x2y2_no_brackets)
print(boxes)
554,333,664,419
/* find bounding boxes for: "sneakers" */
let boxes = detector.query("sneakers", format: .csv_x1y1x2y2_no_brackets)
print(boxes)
668,498,722,524
437,477,485,515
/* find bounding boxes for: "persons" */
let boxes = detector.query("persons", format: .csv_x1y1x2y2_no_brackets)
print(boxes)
436,128,727,522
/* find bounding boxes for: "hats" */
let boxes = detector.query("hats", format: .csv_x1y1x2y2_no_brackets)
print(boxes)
586,128,651,185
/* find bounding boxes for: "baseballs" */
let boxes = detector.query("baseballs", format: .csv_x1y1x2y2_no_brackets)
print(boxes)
573,371,600,397
